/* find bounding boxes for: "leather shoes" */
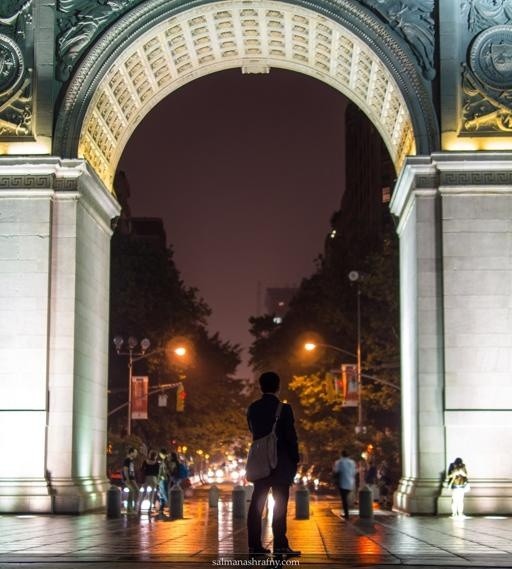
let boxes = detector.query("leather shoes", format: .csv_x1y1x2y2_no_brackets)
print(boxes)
249,545,271,554
273,547,301,555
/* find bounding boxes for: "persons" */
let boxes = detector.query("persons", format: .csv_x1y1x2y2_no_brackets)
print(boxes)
246,371,301,558
358,456,401,507
445,457,469,519
121,446,191,519
333,449,356,519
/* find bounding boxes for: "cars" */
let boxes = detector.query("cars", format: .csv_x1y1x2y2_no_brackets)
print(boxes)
292,457,339,495
206,454,248,482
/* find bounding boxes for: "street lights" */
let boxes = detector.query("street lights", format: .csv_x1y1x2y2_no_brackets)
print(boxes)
112,333,189,461
302,334,379,374
348,268,370,437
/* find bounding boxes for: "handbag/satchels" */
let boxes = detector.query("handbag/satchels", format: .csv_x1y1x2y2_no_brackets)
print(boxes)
246,432,278,481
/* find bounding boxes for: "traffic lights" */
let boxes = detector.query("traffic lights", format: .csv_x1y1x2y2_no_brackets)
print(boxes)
176,388,186,412
